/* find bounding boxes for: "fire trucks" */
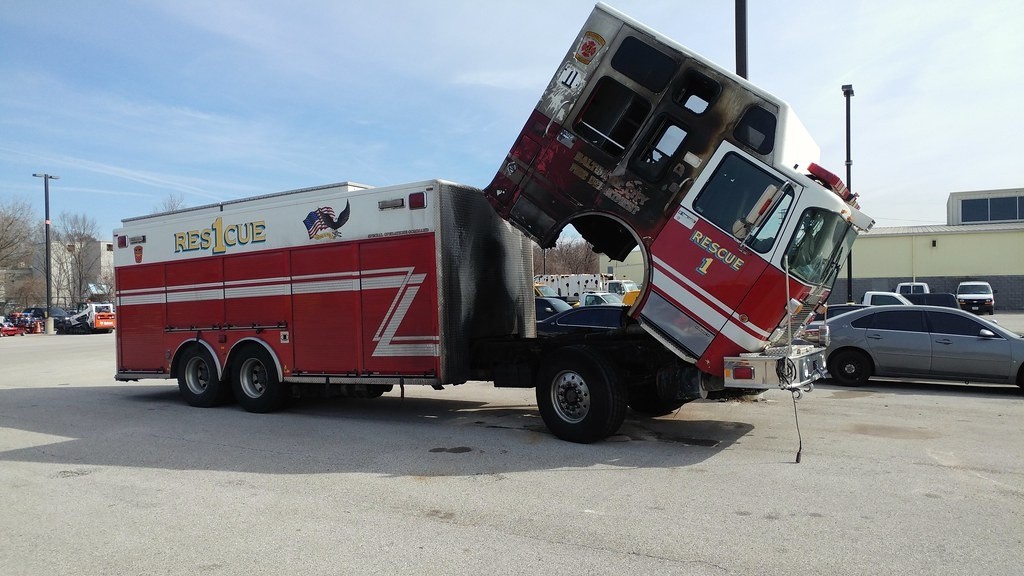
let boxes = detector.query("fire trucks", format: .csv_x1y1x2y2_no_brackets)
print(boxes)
107,1,877,443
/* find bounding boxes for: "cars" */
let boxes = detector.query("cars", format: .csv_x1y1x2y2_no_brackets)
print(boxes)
813,282,960,321
535,297,623,335
952,281,998,315
807,305,1024,390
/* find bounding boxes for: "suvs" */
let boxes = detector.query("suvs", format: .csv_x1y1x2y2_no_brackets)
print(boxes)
21,307,73,333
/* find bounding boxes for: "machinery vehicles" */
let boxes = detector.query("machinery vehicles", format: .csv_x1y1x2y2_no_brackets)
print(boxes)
533,274,642,305
71,303,115,333
